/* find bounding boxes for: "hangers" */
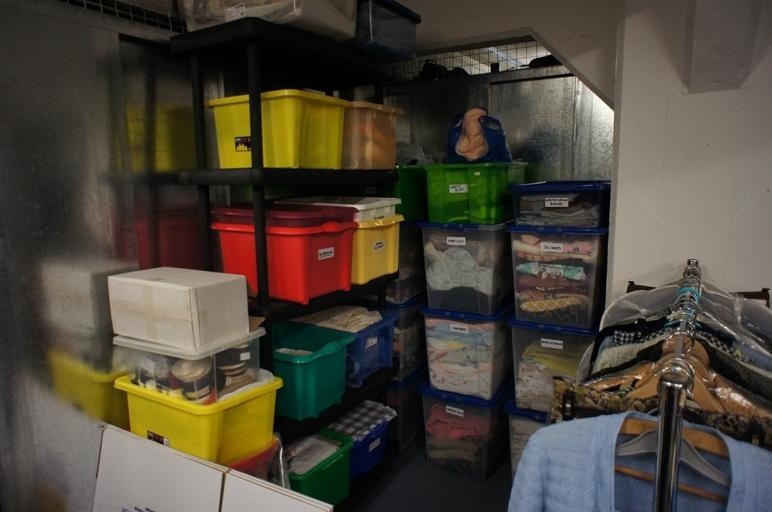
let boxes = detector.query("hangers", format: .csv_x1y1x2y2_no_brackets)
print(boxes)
562,251,771,504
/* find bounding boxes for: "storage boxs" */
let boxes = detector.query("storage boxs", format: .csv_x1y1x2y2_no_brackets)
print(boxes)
109,1,608,512
45,250,137,430
93,97,216,270
